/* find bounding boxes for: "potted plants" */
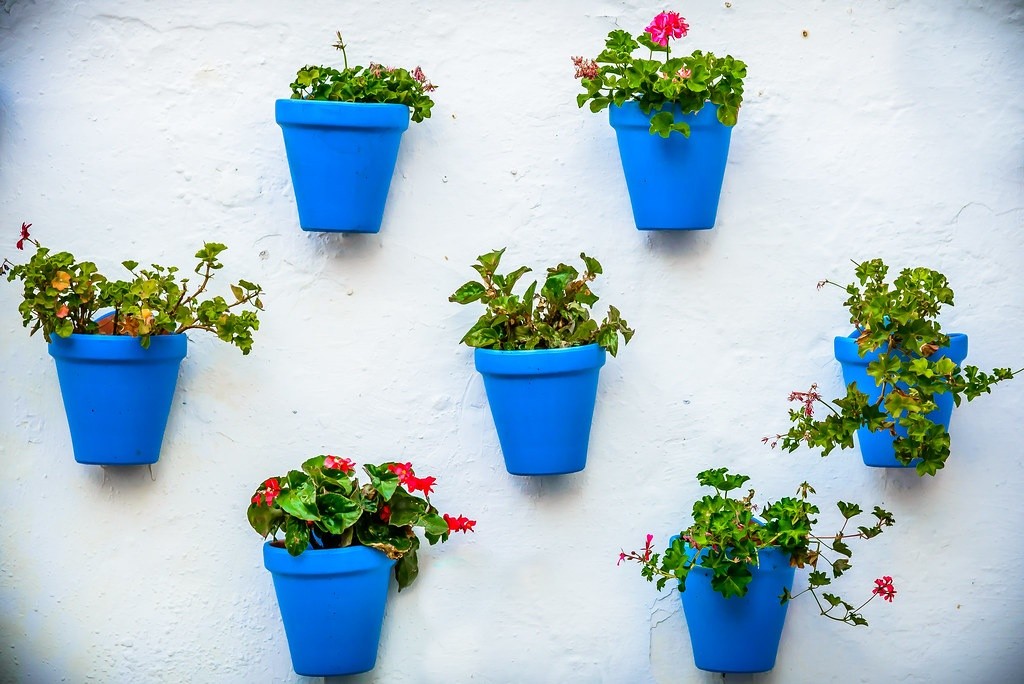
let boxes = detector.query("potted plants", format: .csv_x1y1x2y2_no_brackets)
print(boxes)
448,246,635,475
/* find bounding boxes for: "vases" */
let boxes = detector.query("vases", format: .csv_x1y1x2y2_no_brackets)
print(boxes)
834,316,968,469
262,529,397,678
609,99,732,231
669,519,797,674
48,310,188,466
275,99,410,234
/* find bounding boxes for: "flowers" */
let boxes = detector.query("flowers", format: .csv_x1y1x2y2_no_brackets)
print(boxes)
290,30,438,123
246,454,476,593
0,220,266,356
616,466,897,626
570,8,747,139
761,258,1024,478
